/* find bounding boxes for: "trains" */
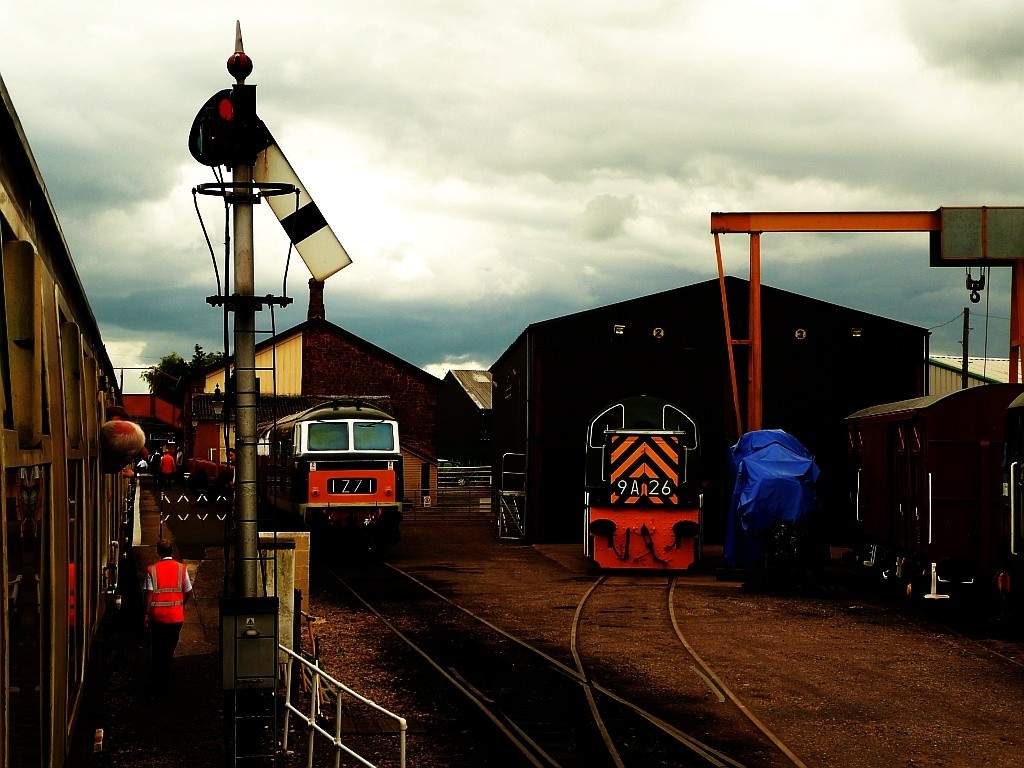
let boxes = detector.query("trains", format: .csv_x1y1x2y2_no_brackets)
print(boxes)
580,396,705,571
842,382,1023,621
256,394,403,545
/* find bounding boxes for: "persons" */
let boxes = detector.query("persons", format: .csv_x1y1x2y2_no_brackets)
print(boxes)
135,446,183,491
143,539,192,682
